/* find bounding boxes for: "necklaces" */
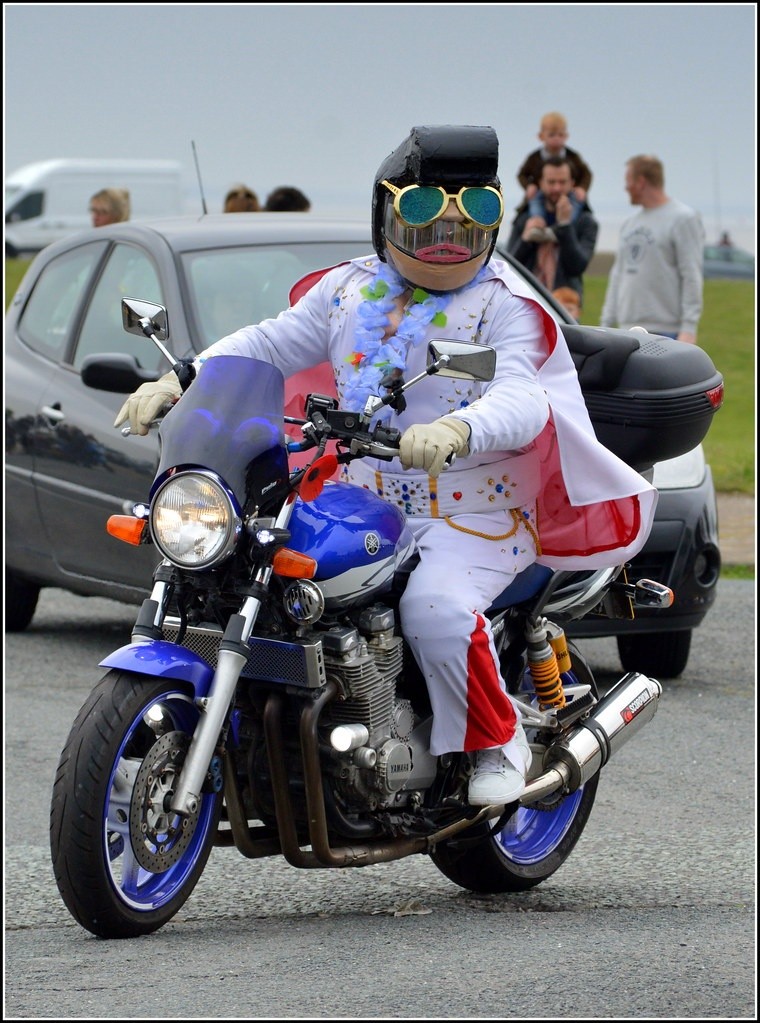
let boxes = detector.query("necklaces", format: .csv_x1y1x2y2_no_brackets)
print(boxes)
338,248,486,433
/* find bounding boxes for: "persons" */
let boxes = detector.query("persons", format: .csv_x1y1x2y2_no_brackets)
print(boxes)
599,153,705,345
507,114,598,326
112,124,658,805
224,184,311,212
90,188,130,226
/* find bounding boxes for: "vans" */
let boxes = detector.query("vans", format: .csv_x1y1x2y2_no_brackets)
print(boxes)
1,154,205,261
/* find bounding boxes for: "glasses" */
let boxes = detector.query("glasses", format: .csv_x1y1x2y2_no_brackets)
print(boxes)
381,180,504,233
88,206,106,216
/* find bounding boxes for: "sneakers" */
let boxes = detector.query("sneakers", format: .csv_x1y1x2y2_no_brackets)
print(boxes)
468,718,535,804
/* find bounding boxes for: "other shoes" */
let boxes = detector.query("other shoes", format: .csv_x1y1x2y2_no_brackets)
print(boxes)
521,216,550,242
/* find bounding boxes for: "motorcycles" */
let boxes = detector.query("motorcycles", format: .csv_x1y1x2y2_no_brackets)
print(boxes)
48,295,724,941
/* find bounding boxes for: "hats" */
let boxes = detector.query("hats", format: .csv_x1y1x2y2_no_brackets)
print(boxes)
91,188,130,225
222,184,256,202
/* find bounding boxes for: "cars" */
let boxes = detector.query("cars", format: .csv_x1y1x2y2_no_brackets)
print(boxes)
5,204,722,682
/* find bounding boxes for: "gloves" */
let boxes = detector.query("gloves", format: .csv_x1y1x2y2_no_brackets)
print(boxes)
396,416,472,478
112,371,184,437
